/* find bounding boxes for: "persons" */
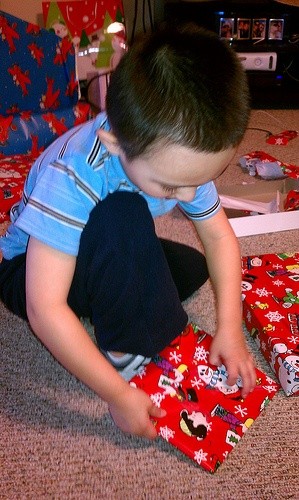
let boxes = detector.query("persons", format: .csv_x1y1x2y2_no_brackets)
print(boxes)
0,23,259,440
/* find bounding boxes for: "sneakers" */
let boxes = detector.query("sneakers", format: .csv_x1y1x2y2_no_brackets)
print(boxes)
103,350,152,383
